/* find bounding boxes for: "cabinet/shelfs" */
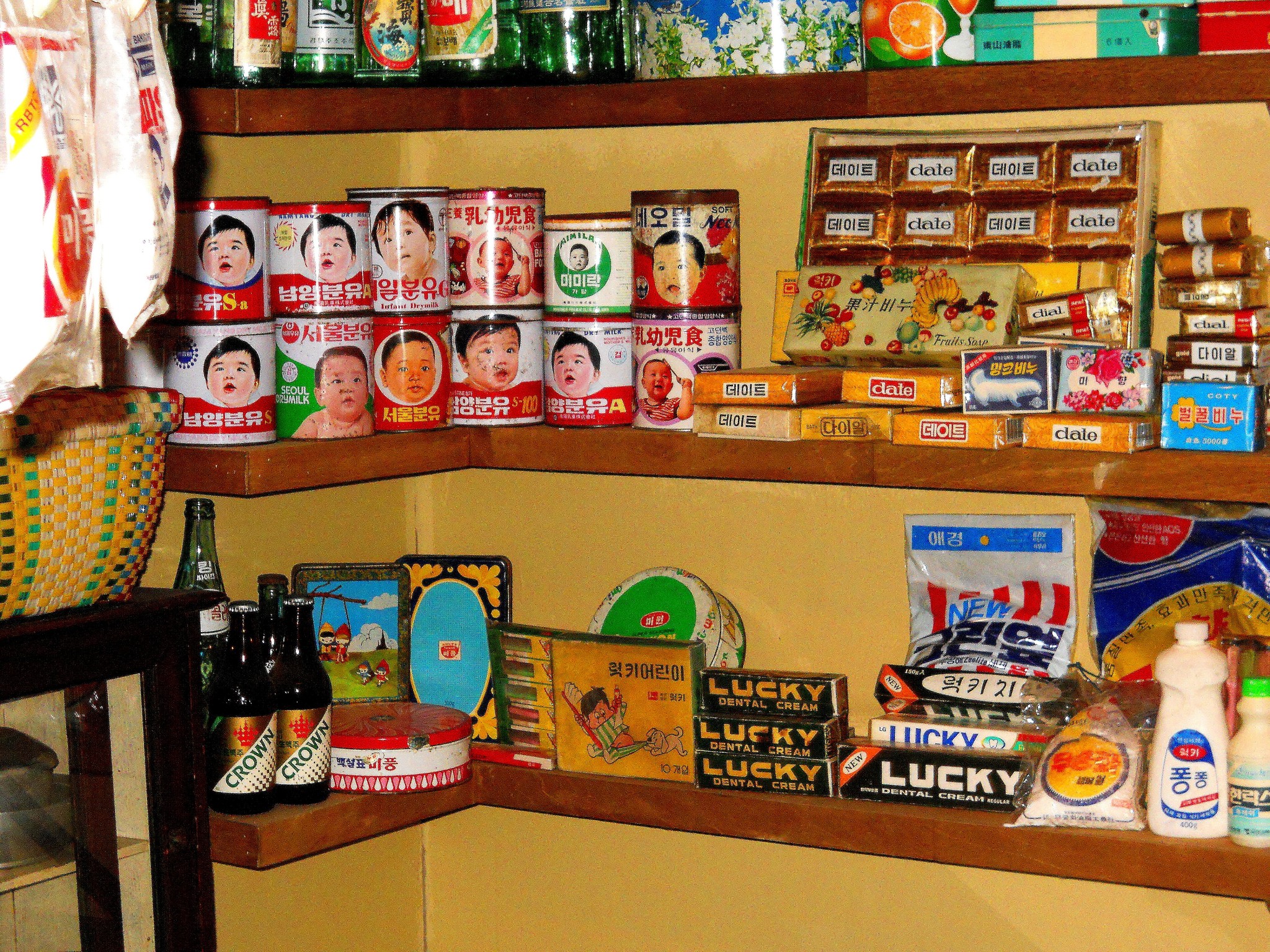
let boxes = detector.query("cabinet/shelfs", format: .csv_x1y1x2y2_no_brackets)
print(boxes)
161,61,1270,908
1,836,151,951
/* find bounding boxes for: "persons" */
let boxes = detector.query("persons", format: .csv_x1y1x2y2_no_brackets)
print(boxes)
289,345,374,437
551,331,601,398
474,237,531,299
203,336,260,409
197,214,256,286
300,214,356,282
640,358,695,422
651,230,707,304
569,244,589,270
379,332,436,402
372,199,446,282
456,315,520,394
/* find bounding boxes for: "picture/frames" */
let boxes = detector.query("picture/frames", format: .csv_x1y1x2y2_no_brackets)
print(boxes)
291,562,412,706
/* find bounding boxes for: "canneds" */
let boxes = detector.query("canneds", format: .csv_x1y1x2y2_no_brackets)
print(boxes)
169,182,741,445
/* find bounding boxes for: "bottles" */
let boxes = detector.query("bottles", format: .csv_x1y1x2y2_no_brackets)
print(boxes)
154,0,634,83
1145,620,1270,849
165,496,333,812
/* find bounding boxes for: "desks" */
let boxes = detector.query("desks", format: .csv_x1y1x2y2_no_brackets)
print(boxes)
1,591,222,952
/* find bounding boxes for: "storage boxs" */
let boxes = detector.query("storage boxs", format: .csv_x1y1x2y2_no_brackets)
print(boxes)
966,5,1198,62
0,386,184,624
1196,1,1269,56
